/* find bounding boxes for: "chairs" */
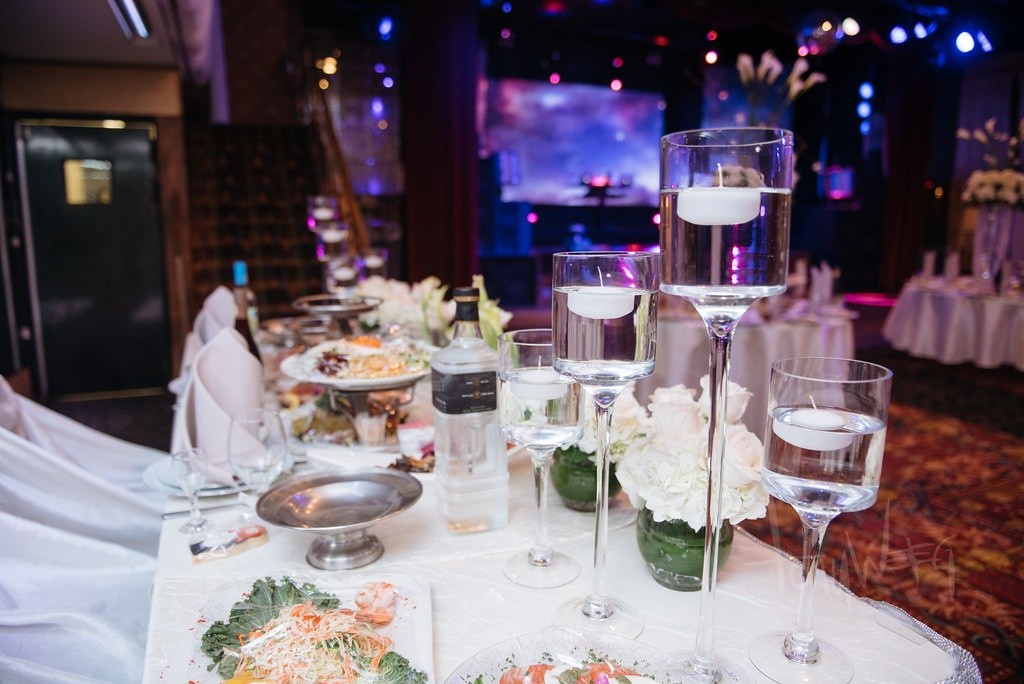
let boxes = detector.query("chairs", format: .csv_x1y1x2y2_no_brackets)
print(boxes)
0,378,170,684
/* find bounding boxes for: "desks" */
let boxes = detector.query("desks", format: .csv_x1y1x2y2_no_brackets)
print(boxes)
143,380,979,684
897,274,1024,402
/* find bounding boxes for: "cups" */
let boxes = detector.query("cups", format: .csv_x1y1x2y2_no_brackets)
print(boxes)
226,411,287,499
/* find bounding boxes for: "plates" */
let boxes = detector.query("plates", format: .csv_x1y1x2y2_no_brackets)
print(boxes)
142,457,248,498
279,340,441,391
143,574,434,684
443,627,712,684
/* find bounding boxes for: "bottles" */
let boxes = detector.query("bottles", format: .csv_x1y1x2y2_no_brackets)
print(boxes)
233,262,261,360
429,288,505,534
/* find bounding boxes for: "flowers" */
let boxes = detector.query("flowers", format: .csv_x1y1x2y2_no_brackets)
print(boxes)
343,275,457,344
474,278,514,362
618,380,768,529
578,385,648,467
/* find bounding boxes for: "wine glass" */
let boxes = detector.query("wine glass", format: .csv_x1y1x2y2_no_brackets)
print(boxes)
171,449,214,534
657,128,794,684
751,357,892,684
499,329,584,586
551,250,657,641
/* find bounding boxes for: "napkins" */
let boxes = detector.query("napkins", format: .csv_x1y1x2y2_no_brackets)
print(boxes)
181,329,264,477
180,285,249,380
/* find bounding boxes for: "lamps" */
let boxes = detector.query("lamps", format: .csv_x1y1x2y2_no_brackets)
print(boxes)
104,0,150,42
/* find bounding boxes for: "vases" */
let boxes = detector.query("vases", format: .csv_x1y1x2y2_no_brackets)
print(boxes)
549,446,621,511
635,512,733,592
